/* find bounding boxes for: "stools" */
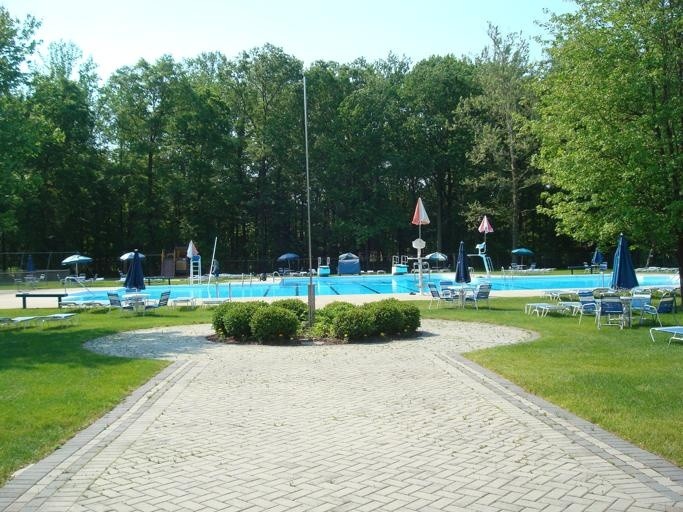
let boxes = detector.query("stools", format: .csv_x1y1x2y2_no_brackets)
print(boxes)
649,325,683,346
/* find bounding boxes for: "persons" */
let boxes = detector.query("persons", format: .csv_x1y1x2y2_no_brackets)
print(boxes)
209,257,220,283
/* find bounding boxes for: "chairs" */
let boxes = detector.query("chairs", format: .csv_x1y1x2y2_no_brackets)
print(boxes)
106,287,173,318
572,287,678,330
428,280,492,310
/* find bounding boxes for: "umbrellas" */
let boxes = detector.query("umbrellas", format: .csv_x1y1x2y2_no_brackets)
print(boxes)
187,239,200,259
119,251,146,260
61,253,93,277
26,255,34,276
455,241,472,287
426,252,449,269
277,253,300,269
609,232,640,290
123,249,145,290
592,248,605,265
512,248,535,264
411,197,430,257
477,215,495,252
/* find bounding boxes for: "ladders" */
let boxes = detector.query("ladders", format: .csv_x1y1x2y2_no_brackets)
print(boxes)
190,255,201,284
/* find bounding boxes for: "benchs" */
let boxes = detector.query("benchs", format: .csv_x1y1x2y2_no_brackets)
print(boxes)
544,289,577,302
0,313,75,331
524,303,568,318
58,301,110,312
173,296,198,309
